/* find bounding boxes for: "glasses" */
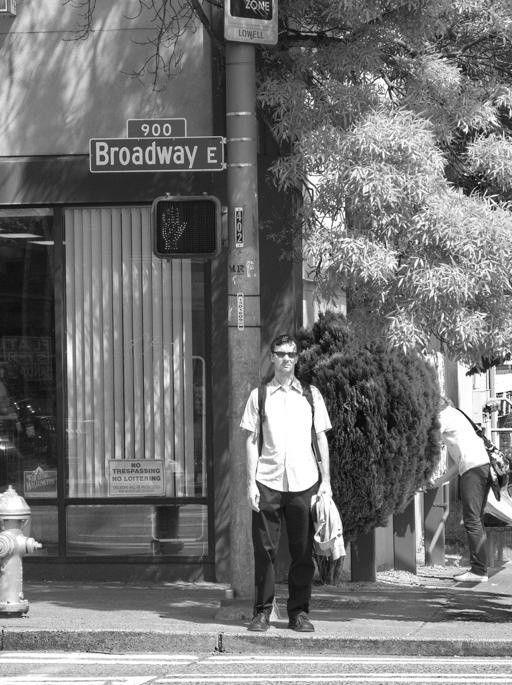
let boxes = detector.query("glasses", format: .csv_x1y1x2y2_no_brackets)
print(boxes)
274,352,297,358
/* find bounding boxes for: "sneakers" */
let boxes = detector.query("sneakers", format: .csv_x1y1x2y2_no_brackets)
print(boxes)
288,611,314,632
247,606,273,632
453,569,488,583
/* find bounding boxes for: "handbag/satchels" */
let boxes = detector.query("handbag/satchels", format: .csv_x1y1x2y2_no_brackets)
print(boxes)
485,446,512,501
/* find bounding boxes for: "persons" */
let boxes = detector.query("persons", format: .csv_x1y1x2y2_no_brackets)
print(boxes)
238,333,334,631
436,398,492,583
